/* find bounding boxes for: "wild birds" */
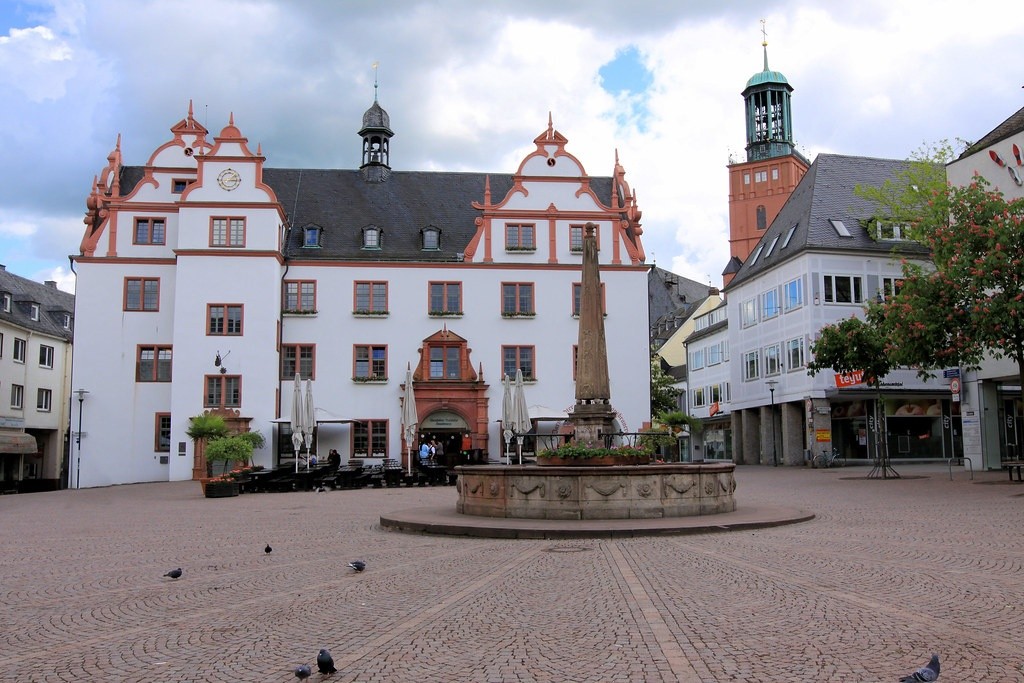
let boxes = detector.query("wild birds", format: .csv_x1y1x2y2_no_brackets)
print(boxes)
162,543,365,683
899,653,941,683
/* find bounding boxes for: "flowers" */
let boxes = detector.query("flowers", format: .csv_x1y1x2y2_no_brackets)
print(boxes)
536,441,652,458
207,464,252,483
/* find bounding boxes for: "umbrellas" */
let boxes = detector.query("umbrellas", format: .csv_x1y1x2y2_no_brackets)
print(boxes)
502,369,533,465
270,408,354,455
291,373,317,473
399,362,418,476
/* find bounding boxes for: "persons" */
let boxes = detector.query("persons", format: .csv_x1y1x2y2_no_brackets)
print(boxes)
419,438,444,465
328,449,341,470
308,451,317,465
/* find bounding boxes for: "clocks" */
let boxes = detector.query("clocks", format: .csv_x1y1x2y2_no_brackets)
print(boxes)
217,168,242,191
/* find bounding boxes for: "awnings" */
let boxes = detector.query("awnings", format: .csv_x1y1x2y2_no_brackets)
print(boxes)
0,431,38,453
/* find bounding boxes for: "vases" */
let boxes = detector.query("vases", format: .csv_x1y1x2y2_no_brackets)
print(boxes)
205,483,239,498
537,455,650,466
